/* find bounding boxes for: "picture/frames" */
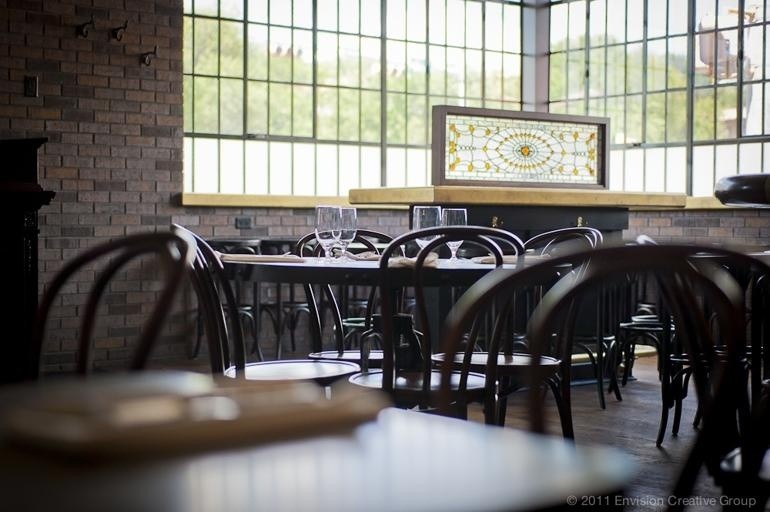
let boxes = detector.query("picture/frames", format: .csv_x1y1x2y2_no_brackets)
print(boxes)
430,104,611,191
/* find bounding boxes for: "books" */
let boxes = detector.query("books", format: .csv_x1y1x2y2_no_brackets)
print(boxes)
216,250,305,263
471,256,547,264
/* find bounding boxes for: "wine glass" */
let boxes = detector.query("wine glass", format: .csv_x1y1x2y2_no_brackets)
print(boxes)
330,207,359,261
411,205,443,258
443,208,469,267
315,206,342,260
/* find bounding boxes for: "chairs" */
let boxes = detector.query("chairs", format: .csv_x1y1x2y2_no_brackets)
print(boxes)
2,204,768,512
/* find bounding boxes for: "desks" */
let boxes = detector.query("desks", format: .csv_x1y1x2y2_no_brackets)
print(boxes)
347,185,685,355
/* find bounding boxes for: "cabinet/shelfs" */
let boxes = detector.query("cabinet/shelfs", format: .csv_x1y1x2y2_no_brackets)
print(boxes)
0,133,57,382
